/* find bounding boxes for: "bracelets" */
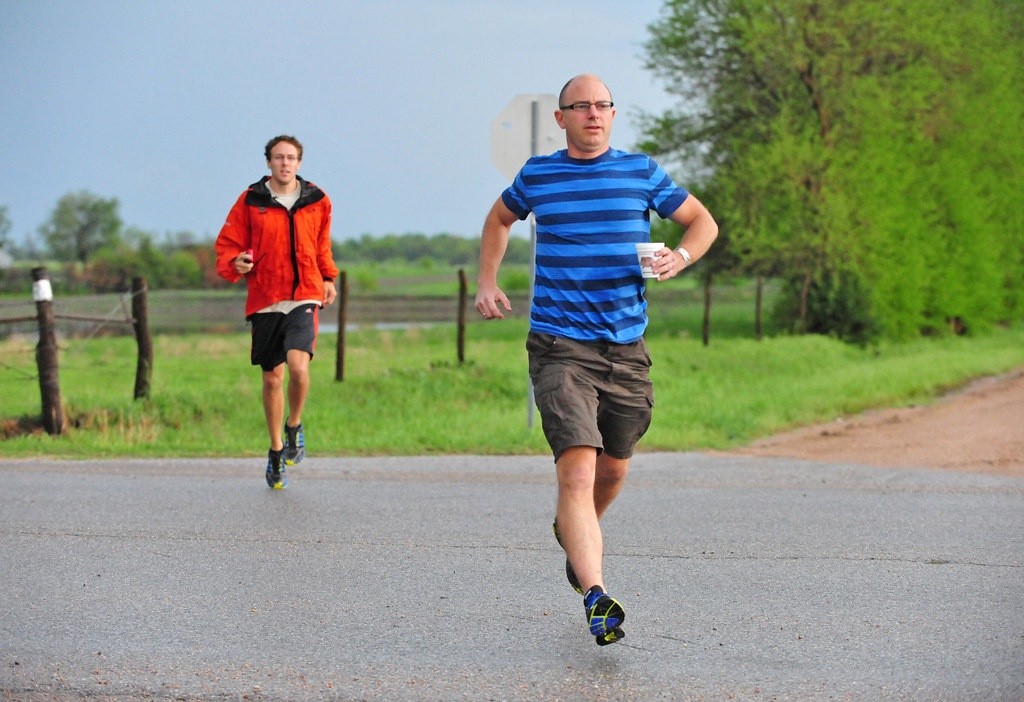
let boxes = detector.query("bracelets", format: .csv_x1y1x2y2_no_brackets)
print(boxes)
673,246,692,266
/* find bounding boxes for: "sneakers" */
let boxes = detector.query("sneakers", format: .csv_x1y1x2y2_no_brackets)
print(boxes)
283,417,305,466
583,585,625,645
266,447,289,490
554,518,587,596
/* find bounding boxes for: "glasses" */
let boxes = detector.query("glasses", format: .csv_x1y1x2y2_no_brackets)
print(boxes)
561,100,614,111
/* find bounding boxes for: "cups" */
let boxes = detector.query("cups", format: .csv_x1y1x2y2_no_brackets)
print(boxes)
635,242,665,278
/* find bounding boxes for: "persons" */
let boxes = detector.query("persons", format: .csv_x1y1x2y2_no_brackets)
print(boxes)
474,74,718,645
215,135,339,488
